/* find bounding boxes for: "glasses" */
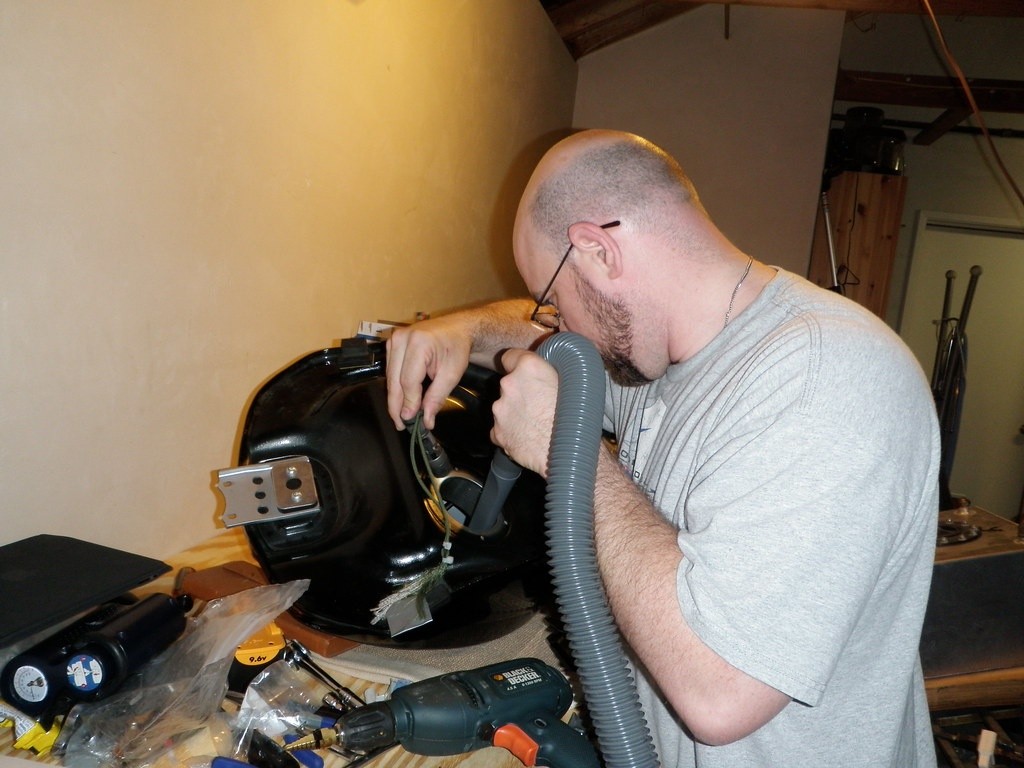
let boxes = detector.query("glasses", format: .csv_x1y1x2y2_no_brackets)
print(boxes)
531,220,620,328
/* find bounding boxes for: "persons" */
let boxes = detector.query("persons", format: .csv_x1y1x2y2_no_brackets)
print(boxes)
385,129,943,767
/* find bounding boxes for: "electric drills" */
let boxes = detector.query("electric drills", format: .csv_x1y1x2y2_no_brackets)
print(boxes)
282,656,603,768
